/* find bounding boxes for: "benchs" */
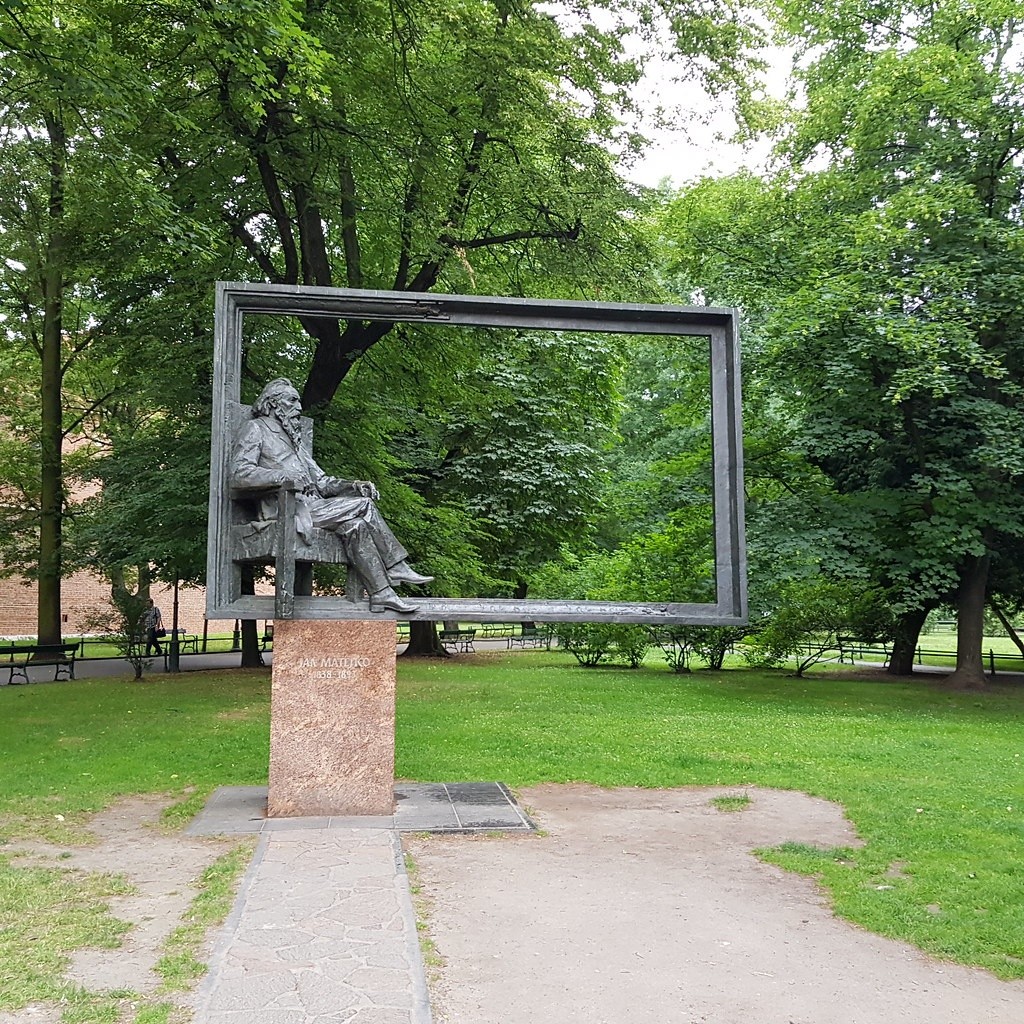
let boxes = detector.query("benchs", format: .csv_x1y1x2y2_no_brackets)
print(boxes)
837,636,895,669
480,621,514,638
0,643,79,686
155,628,197,654
439,629,476,654
260,625,274,652
507,627,547,649
396,624,411,644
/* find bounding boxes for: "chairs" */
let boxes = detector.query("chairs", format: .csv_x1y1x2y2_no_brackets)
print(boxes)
216,401,365,619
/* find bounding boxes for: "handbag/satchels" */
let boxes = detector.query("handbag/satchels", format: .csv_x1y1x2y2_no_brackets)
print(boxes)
157,628,166,637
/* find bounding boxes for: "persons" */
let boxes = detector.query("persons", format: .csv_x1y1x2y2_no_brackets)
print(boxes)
138,598,162,656
229,377,434,612
